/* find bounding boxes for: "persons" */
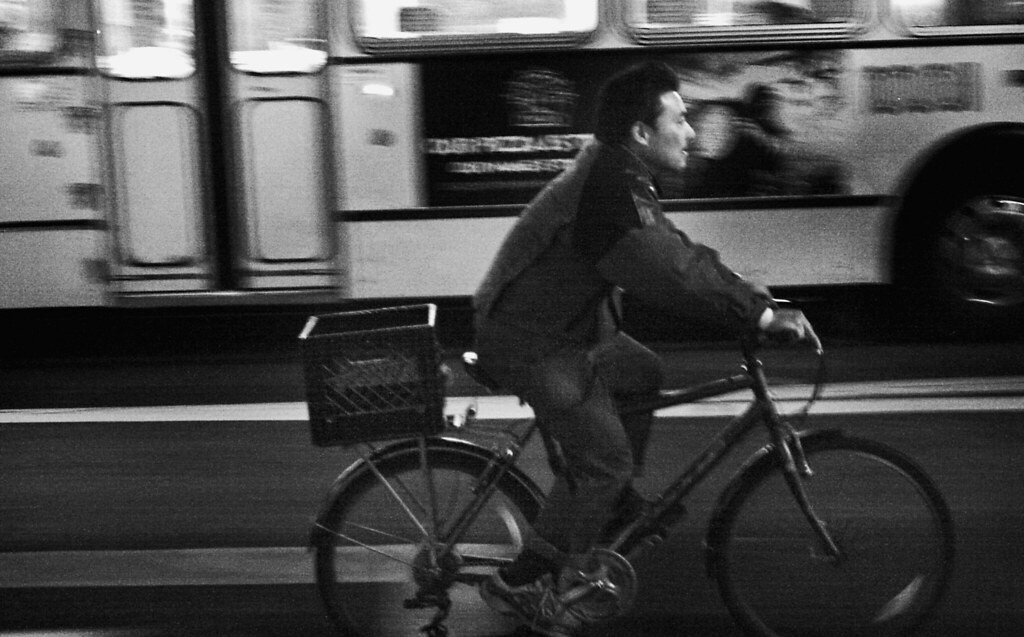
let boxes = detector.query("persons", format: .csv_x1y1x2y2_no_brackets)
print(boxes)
400,7,437,31
474,68,822,637
750,1,814,25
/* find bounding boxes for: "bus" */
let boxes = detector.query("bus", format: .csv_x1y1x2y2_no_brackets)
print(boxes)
0,0,1024,336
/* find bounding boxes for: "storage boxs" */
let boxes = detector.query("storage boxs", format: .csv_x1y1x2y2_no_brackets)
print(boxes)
300,301,438,440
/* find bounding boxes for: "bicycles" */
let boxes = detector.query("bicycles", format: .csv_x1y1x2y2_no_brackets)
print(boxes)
303,295,959,637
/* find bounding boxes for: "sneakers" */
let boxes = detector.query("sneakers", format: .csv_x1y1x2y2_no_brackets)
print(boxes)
593,485,689,556
478,566,583,637
